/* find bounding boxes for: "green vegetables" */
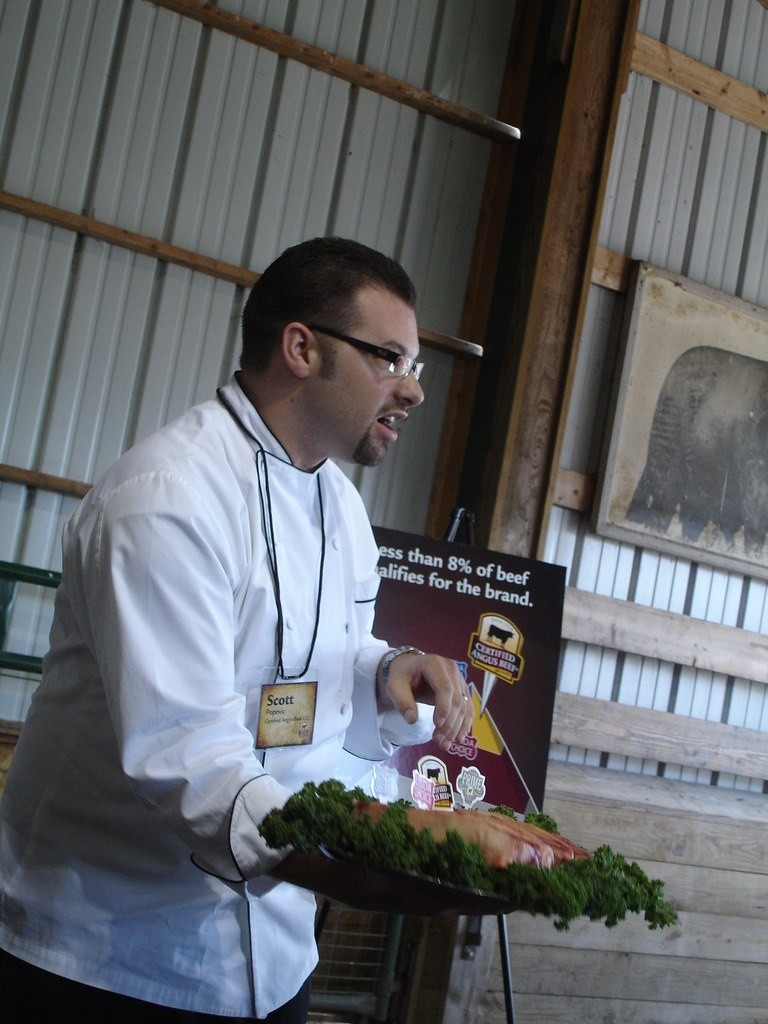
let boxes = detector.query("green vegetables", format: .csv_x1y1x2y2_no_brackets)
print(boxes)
258,779,680,929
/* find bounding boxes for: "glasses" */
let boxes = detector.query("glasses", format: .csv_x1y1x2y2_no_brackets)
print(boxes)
306,323,425,381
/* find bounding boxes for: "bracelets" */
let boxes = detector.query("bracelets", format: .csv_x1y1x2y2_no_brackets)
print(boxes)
382,645,425,685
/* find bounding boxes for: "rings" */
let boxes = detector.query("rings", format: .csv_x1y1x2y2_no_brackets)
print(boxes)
462,693,468,701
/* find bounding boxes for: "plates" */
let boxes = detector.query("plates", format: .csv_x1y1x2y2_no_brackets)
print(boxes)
321,846,519,914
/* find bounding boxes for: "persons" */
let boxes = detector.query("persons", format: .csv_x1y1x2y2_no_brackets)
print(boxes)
0,236,473,1024
